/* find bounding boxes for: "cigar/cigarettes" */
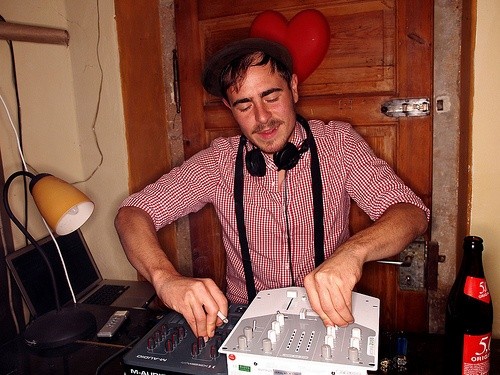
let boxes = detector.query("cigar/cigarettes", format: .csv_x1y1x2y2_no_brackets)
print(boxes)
216,310,229,324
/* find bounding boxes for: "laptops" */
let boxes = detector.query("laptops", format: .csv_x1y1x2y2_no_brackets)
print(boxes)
4,228,157,309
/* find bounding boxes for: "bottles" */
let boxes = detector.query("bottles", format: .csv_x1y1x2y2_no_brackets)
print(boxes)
441,236,493,375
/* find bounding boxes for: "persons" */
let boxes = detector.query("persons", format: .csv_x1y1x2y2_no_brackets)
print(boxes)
115,39,431,342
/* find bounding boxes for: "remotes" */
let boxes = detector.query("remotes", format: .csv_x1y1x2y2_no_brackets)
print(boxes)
98,310,130,337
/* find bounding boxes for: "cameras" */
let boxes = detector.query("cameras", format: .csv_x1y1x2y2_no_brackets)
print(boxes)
244,130,311,177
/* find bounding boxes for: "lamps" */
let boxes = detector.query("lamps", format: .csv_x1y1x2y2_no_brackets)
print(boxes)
3,170,97,348
